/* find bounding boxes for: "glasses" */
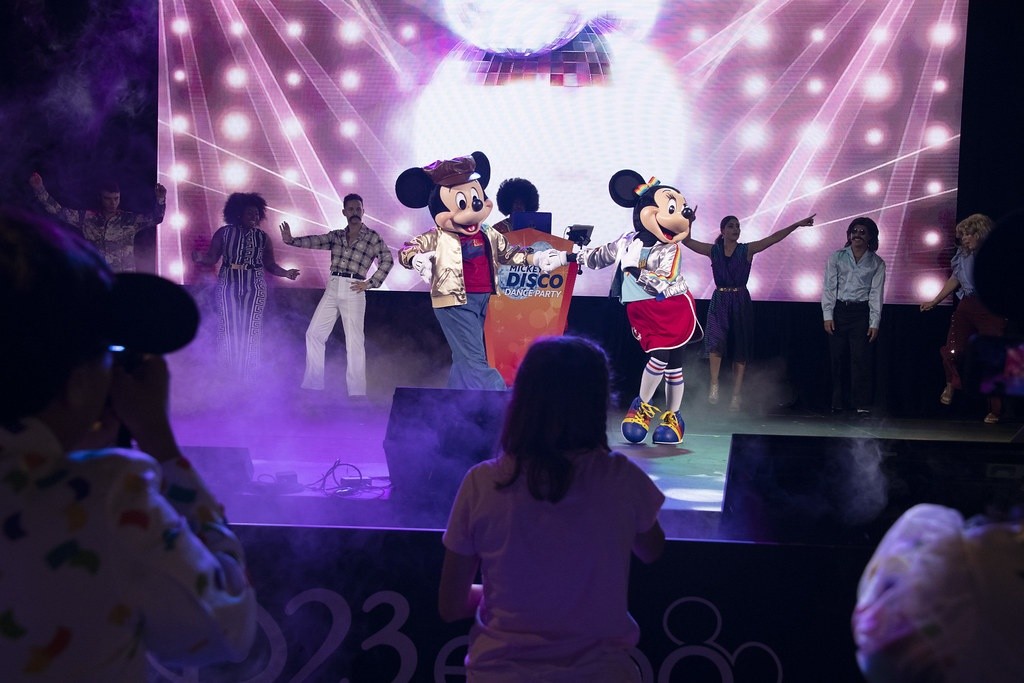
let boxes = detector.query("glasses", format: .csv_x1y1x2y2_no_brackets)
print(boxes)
851,227,869,235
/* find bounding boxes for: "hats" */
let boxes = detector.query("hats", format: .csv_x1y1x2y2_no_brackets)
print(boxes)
853,505,1024,683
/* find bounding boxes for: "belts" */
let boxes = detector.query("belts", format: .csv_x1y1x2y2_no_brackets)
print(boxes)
716,285,745,292
332,272,364,279
837,299,869,307
223,262,256,270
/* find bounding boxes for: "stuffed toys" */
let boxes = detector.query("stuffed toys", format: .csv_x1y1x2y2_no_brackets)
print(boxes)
395,151,557,391
541,169,704,444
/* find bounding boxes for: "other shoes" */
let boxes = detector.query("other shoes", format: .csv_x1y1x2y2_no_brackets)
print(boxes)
941,381,952,405
857,406,870,419
353,404,376,426
985,411,999,424
832,404,844,420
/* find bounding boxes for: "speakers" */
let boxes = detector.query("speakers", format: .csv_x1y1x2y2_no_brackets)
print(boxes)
383,388,513,509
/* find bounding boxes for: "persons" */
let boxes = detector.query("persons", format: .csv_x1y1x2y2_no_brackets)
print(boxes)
279,193,395,401
29,172,166,273
918,213,1006,425
681,204,816,412
0,200,254,683
191,193,301,386
493,178,540,234
437,334,668,683
819,217,887,417
851,501,1024,683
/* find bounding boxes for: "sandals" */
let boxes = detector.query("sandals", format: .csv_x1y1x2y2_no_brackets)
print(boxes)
708,380,720,405
729,396,742,416
